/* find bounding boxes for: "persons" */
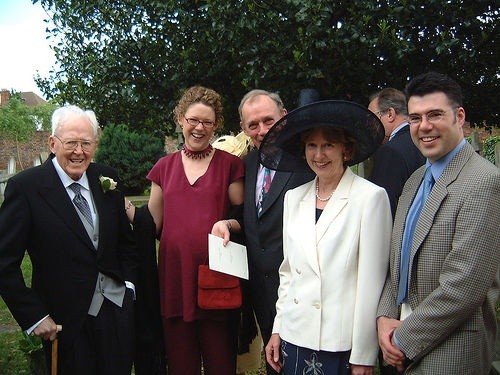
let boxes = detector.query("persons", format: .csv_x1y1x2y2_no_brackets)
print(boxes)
375,72,500,375
0,105,141,375
239,89,316,375
366,88,428,222
258,97,392,375
124,87,246,375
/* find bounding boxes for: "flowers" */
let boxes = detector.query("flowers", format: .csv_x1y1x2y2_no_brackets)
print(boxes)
99,175,119,195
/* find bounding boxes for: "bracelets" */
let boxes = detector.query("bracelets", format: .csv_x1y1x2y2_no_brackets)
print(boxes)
223,220,232,228
125,201,132,211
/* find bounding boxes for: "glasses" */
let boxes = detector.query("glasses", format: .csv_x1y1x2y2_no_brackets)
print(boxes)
182,114,217,129
408,107,459,123
53,135,97,150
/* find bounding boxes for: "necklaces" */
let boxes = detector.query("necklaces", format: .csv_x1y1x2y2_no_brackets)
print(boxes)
180,142,213,159
316,179,337,201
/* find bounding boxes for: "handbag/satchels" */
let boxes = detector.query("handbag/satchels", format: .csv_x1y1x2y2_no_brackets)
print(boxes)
197,264,242,311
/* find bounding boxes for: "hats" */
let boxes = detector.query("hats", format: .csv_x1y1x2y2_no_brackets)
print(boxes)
257,83,386,174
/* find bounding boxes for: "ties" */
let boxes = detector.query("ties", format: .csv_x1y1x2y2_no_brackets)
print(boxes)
395,166,434,305
68,182,94,229
255,168,272,219
